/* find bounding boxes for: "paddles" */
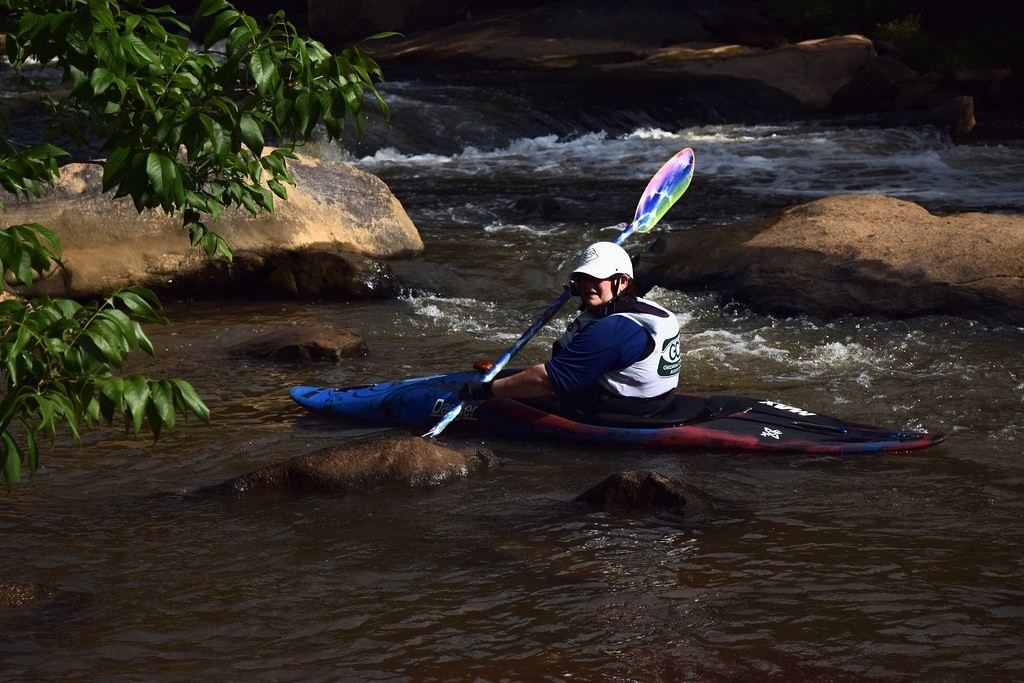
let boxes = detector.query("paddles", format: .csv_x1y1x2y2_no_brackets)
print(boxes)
414,147,697,442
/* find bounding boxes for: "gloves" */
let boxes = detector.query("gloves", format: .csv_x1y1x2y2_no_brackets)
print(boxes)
459,381,491,398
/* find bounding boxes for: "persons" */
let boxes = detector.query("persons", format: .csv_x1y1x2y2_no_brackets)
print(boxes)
458,242,680,416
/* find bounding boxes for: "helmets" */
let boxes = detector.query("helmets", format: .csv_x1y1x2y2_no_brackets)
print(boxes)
572,241,633,280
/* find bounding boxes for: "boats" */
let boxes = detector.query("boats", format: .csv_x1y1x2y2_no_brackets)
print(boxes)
289,377,949,456
